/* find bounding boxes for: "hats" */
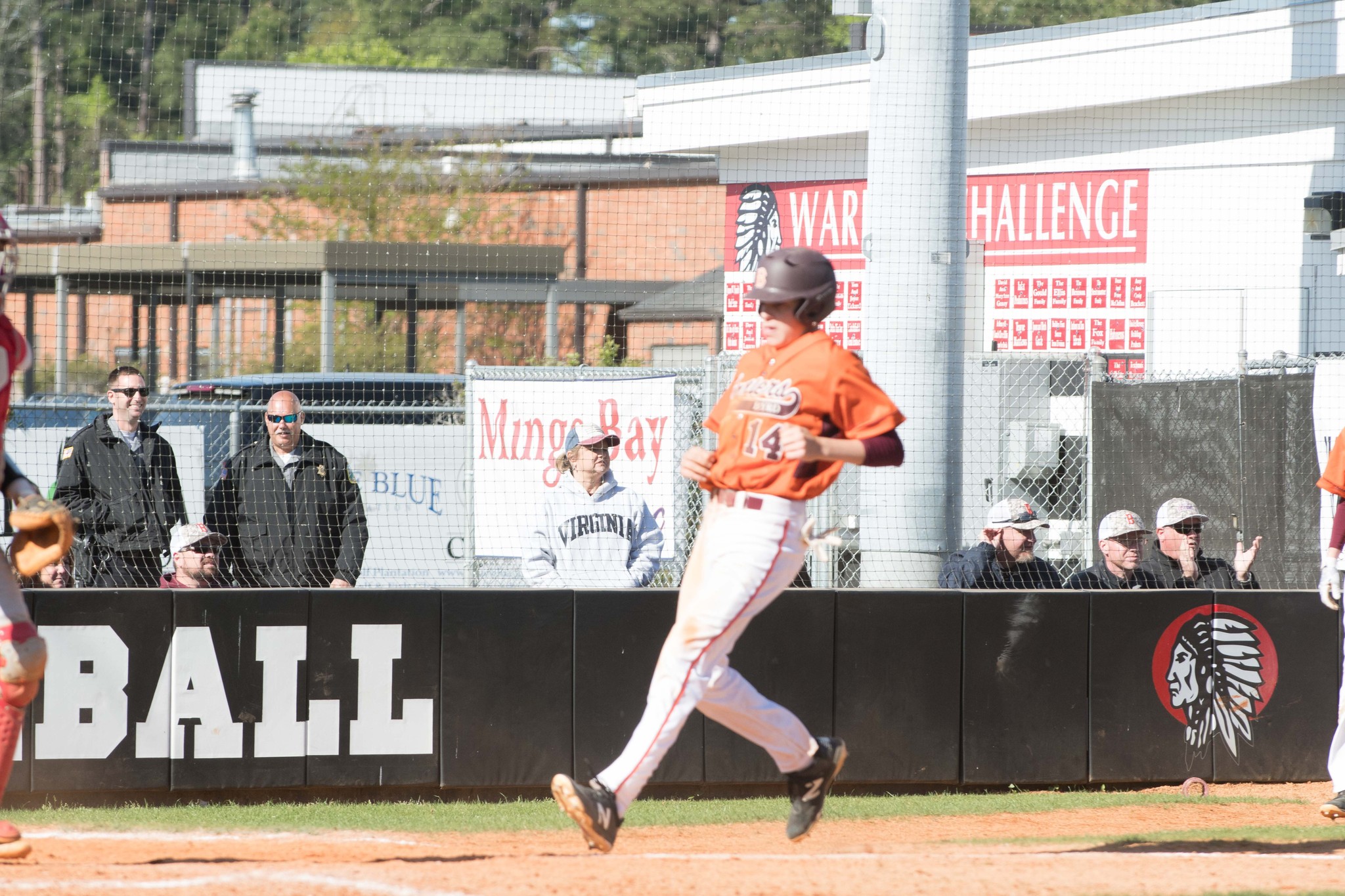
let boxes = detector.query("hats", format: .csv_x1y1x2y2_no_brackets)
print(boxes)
1097,511,1153,539
562,426,620,457
1156,498,1210,533
985,499,1051,531
169,522,228,554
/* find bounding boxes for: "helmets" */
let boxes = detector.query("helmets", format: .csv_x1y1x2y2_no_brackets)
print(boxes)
740,246,835,328
0,217,19,317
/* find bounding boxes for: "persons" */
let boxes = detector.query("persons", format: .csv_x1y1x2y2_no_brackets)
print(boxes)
1064,510,1165,589
552,246,907,854
1138,498,1262,589
0,215,74,858
203,390,369,588
1316,427,1345,819
53,366,181,587
159,523,238,588
521,421,665,587
938,499,1062,589
15,548,73,588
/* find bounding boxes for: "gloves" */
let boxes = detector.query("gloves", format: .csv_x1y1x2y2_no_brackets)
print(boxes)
1317,558,1340,610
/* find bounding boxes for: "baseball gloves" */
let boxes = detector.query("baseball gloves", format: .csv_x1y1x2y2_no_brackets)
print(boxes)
9,491,82,579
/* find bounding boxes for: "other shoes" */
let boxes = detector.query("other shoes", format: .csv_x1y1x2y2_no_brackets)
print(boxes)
0,819,20,843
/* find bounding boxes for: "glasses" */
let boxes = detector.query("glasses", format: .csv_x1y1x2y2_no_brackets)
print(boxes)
1110,536,1149,547
112,387,151,398
1165,522,1205,535
991,508,1037,526
176,542,223,557
267,410,302,424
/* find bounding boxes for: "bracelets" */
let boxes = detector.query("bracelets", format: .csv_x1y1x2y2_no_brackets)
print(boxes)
1236,572,1252,582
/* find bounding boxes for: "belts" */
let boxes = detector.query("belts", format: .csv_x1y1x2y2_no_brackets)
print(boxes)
707,490,763,511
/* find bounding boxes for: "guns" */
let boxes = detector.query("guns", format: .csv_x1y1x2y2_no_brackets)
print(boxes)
71,530,96,583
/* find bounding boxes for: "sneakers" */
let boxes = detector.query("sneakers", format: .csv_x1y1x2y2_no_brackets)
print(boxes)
1321,791,1345,821
550,770,623,855
783,735,847,845
